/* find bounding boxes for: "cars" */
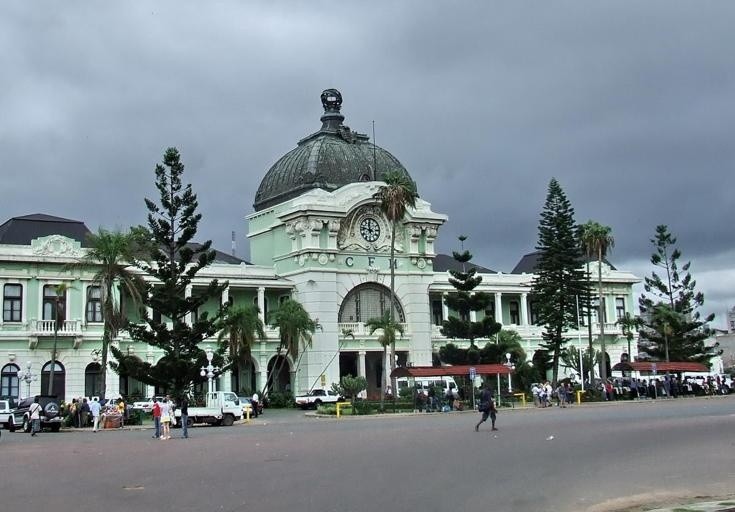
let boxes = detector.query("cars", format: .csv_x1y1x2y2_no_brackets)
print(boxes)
0,391,265,432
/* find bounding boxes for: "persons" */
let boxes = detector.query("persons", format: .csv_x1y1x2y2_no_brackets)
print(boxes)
605,375,726,401
251,390,259,418
28,397,43,436
417,383,509,431
531,380,574,408
70,396,128,432
151,394,188,440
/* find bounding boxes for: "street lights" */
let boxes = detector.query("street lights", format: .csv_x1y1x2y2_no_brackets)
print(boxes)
200,344,220,392
16,360,38,397
502,352,516,393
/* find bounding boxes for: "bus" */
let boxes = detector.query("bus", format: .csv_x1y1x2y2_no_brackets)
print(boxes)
395,375,460,400
680,371,735,394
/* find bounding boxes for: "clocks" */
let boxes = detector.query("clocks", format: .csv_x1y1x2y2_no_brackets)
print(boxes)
359,217,381,243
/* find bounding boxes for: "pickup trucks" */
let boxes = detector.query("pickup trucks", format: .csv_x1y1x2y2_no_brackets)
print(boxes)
295,387,346,410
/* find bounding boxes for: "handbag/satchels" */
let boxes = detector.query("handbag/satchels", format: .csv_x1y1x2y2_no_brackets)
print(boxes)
478,399,491,412
